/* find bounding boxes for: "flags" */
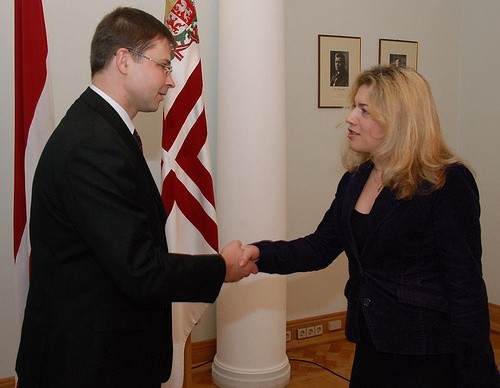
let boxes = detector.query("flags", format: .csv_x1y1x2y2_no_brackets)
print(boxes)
13,0,55,321
160,0,218,388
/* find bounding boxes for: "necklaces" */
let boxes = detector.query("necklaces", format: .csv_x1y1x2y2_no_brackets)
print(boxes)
377,185,382,192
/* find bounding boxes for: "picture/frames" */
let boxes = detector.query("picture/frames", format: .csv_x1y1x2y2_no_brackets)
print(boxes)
318,34,362,109
378,38,419,72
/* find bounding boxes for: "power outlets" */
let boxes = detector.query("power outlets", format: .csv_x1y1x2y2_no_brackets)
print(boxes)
286,331,291,342
306,326,314,338
327,319,342,331
296,328,306,340
314,324,324,336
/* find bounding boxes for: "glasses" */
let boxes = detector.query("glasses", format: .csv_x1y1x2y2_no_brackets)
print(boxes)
115,49,173,76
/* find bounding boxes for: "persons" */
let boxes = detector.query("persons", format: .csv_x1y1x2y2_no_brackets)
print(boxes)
330,53,349,86
239,65,500,388
13,8,258,388
